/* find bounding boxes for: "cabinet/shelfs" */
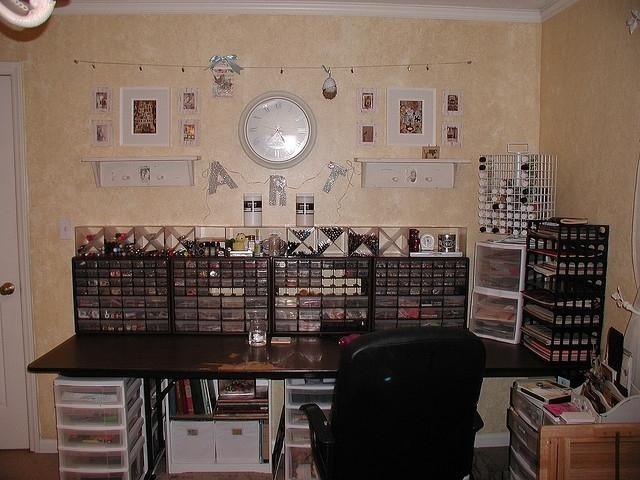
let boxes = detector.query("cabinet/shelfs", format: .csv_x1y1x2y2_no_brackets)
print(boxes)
504,373,640,479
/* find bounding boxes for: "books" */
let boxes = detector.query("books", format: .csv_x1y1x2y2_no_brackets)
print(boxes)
601,362,617,405
169,379,213,416
543,404,595,424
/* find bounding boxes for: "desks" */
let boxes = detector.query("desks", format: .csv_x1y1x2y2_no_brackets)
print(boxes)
24,334,593,479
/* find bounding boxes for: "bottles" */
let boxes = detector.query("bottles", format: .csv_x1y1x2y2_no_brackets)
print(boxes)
410,229,420,252
479,154,552,236
205,241,221,257
268,229,280,258
248,319,267,348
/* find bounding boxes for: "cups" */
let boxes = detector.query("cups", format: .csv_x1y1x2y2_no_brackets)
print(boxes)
247,235,255,256
295,192,315,226
244,194,262,228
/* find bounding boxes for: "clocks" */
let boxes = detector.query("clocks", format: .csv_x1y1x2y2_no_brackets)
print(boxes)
238,90,317,171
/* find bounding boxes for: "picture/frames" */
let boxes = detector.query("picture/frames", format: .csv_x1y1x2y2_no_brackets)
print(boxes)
354,86,463,151
90,86,202,148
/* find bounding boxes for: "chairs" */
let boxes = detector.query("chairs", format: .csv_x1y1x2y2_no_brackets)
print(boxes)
297,326,489,480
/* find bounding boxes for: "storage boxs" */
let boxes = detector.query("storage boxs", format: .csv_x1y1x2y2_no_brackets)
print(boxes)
70,218,610,365
169,418,264,467
